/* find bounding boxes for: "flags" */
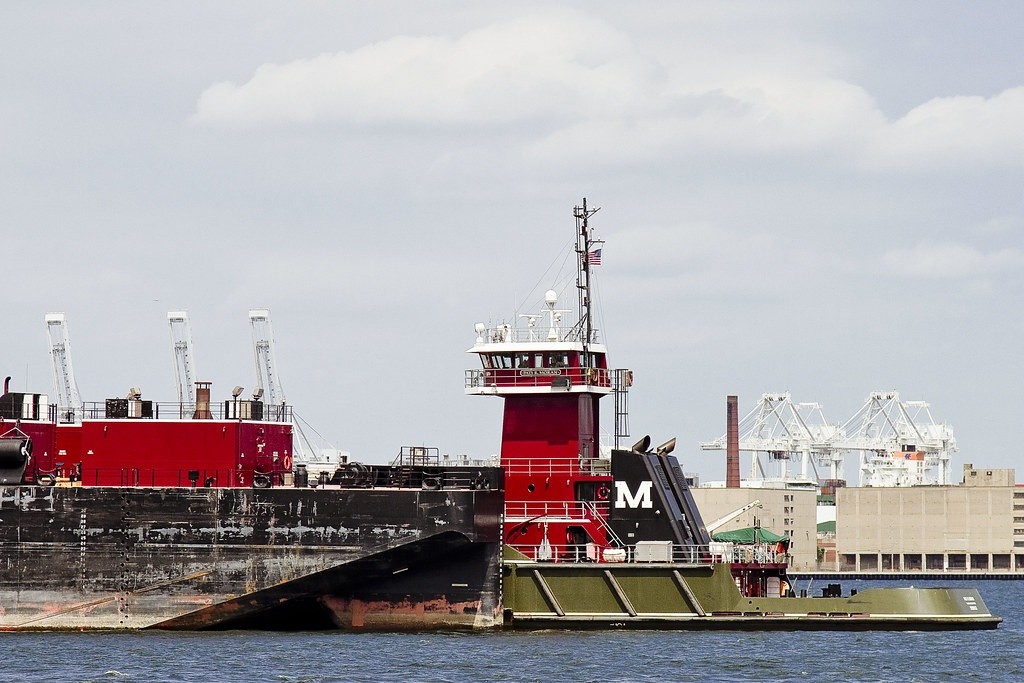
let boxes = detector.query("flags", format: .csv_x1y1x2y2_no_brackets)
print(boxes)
581,249,602,267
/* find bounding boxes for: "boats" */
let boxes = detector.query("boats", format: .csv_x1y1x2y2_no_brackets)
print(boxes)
698,389,959,466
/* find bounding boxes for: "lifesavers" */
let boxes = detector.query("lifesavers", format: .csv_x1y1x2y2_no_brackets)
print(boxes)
284,456,290,469
567,531,573,543
597,486,609,500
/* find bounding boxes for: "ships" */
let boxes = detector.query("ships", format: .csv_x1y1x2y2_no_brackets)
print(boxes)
1,195,1004,635
